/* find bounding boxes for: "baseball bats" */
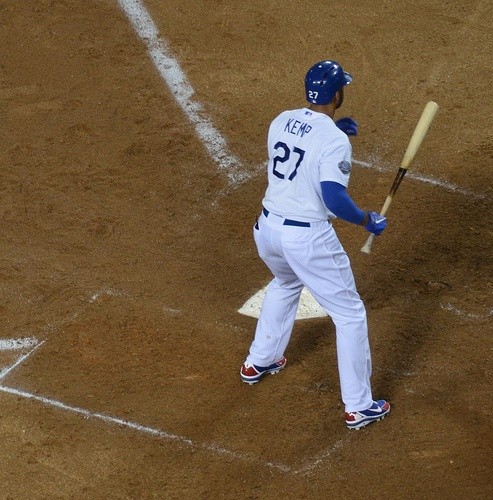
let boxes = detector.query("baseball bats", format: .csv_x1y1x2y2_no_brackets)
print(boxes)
360,101,439,256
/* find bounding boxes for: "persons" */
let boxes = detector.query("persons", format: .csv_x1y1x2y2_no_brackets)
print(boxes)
239,61,391,430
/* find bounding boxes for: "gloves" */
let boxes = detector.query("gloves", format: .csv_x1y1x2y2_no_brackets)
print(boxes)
365,211,387,235
334,117,358,137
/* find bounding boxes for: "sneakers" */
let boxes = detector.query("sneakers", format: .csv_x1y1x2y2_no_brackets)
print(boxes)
239,356,287,384
343,400,390,429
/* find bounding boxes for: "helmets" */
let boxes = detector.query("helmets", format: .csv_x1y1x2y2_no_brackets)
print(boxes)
304,61,353,106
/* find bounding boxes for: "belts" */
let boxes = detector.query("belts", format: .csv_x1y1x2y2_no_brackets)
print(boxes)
261,206,331,228
